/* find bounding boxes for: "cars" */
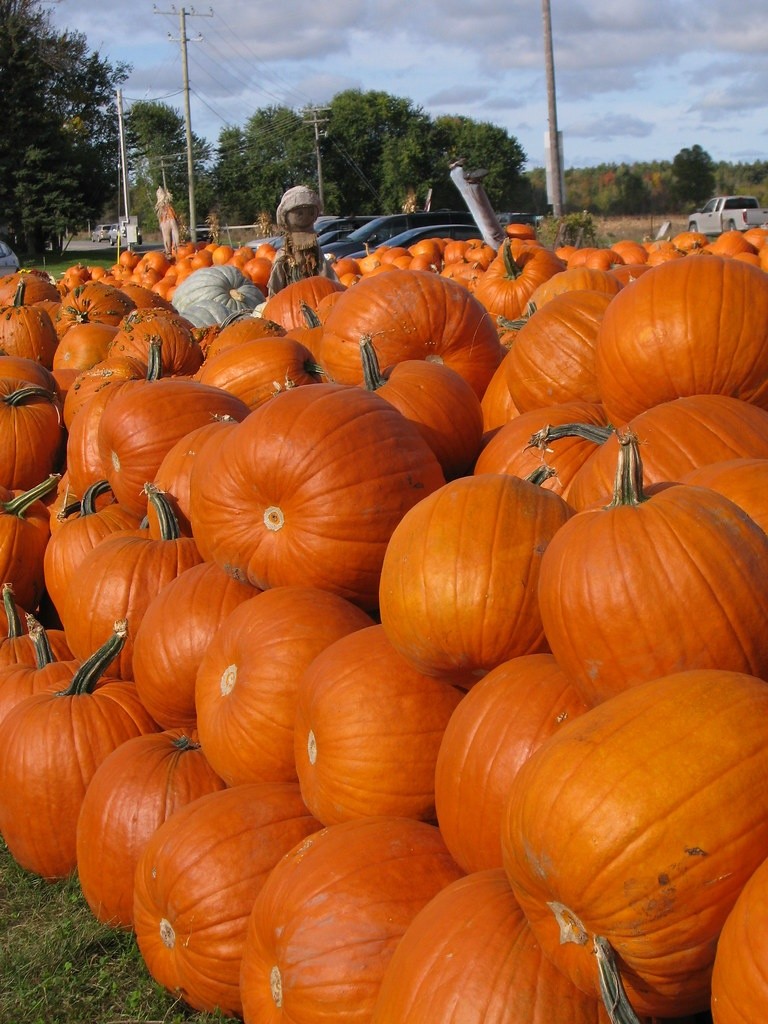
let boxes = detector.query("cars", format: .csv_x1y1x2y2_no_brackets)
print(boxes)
244,214,392,255
91,225,112,242
0,240,21,277
108,223,143,246
344,224,484,261
182,223,213,242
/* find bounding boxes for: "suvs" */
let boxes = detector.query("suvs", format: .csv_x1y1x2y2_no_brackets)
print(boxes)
497,211,539,229
319,208,480,264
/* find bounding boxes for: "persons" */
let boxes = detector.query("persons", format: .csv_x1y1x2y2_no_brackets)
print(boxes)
155,186,182,260
266,185,342,300
447,153,508,251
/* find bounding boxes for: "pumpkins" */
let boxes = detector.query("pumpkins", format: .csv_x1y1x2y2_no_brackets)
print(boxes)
0,223,768,1024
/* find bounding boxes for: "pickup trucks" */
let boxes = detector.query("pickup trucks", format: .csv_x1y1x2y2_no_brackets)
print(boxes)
688,195,768,237
